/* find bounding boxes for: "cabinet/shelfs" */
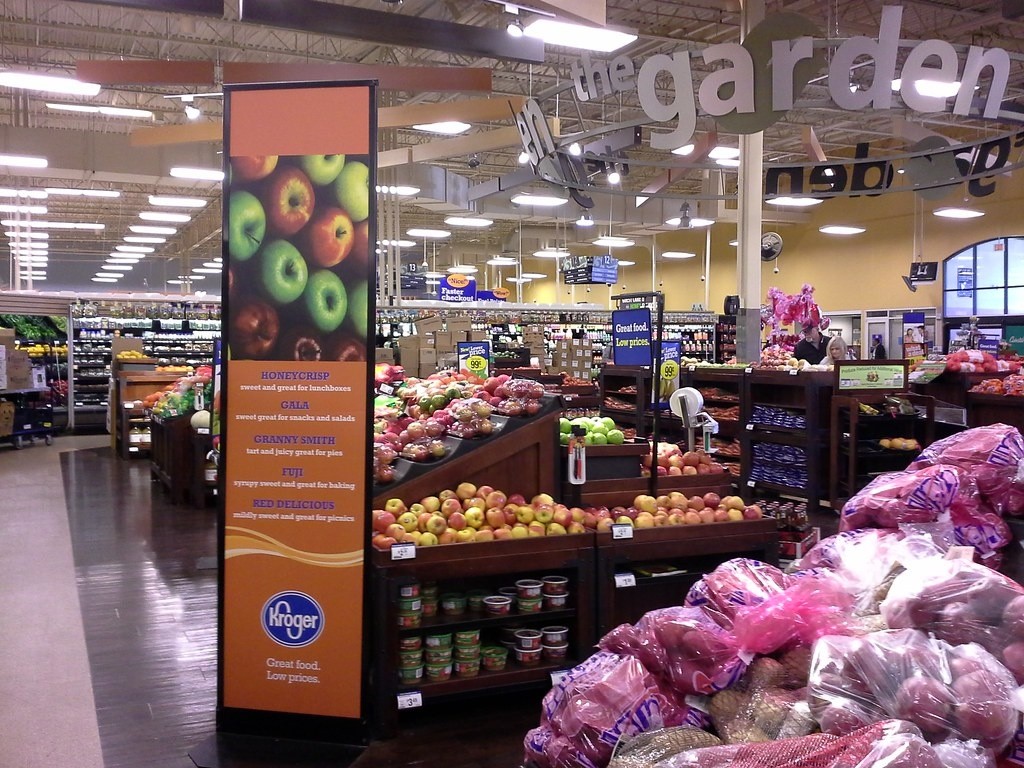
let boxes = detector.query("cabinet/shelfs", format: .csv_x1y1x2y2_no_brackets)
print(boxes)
716,321,737,363
372,529,595,740
191,430,219,509
599,364,835,513
69,298,222,434
0,339,71,435
375,307,716,365
830,390,937,509
112,367,197,460
588,513,780,652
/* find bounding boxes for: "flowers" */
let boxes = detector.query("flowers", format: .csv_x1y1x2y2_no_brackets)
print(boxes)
957,316,987,338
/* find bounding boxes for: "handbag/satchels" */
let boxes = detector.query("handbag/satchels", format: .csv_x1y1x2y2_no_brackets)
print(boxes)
521,422,1024,768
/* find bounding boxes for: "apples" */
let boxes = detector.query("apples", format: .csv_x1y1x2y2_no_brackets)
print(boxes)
806,565,1024,757
224,154,369,362
140,370,212,419
370,366,761,551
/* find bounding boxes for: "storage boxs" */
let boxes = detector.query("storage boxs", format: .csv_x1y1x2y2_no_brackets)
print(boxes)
560,438,650,481
376,348,395,366
0,402,15,435
914,398,968,426
14,407,52,430
398,315,487,378
106,378,114,433
0,327,46,390
522,327,545,373
547,339,593,381
562,385,595,395
117,358,158,372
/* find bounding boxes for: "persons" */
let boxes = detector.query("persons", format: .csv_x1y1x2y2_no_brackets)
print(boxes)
820,335,857,366
870,337,886,361
793,317,831,365
904,328,914,343
913,325,924,343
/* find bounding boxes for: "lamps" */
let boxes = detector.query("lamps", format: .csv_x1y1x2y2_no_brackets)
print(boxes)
0,186,48,199
405,227,451,238
0,203,48,214
510,194,569,206
676,199,693,231
506,18,525,37
444,216,493,226
0,69,101,95
574,190,594,226
0,153,48,168
819,225,866,235
932,207,986,219
522,14,640,53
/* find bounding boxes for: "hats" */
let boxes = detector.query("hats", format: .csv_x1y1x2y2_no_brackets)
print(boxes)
800,318,818,334
